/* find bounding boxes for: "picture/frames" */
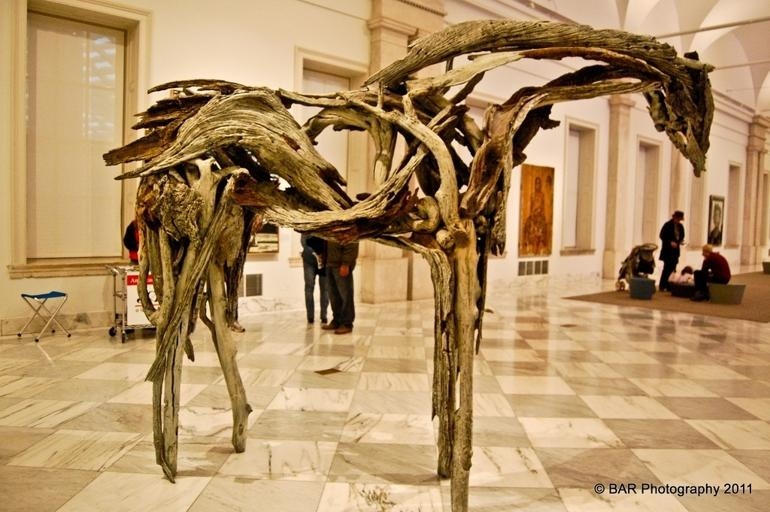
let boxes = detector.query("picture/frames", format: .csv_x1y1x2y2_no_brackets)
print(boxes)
706,194,727,247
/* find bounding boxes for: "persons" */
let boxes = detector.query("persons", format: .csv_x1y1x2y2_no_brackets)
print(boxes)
658,210,684,292
711,201,721,242
321,232,357,336
522,177,547,255
689,243,731,303
299,233,331,330
126,214,137,264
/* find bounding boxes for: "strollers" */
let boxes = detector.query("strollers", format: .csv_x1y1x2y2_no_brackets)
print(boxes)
614,242,660,292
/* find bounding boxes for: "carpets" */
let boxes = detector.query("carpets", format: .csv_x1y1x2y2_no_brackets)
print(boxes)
563,270,769,323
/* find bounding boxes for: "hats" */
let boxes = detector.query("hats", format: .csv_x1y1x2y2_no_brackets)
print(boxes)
673,210,685,222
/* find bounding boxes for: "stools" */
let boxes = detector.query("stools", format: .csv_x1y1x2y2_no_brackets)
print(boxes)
15,291,73,343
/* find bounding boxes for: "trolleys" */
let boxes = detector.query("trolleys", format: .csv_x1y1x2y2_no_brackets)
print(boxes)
103,261,160,345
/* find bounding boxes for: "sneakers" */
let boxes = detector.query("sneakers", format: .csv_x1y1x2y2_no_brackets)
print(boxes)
306,322,334,331
334,326,351,334
